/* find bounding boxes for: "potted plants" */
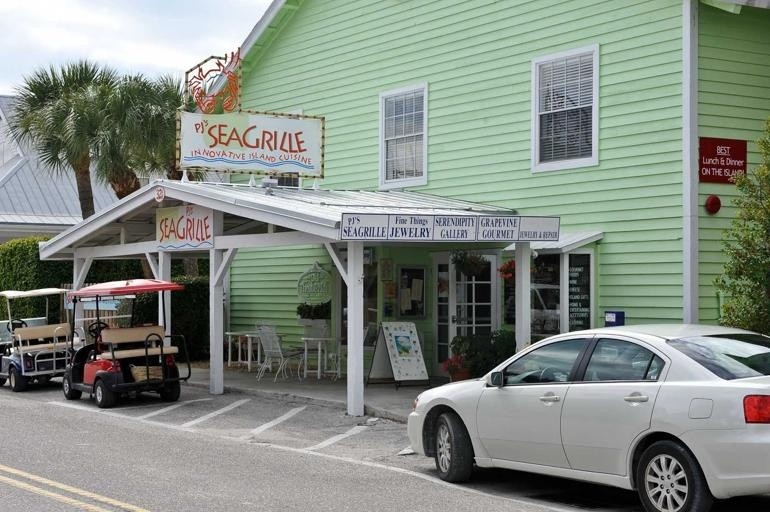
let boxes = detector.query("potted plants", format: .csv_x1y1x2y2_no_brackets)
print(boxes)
445,329,516,382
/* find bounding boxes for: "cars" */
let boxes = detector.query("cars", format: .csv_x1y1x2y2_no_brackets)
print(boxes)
406,323,770,512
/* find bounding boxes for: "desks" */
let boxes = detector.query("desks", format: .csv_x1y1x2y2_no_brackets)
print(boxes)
300,337,344,381
225,331,286,373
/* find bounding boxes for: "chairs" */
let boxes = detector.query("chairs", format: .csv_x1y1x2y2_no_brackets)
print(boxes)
301,319,339,378
255,326,304,384
